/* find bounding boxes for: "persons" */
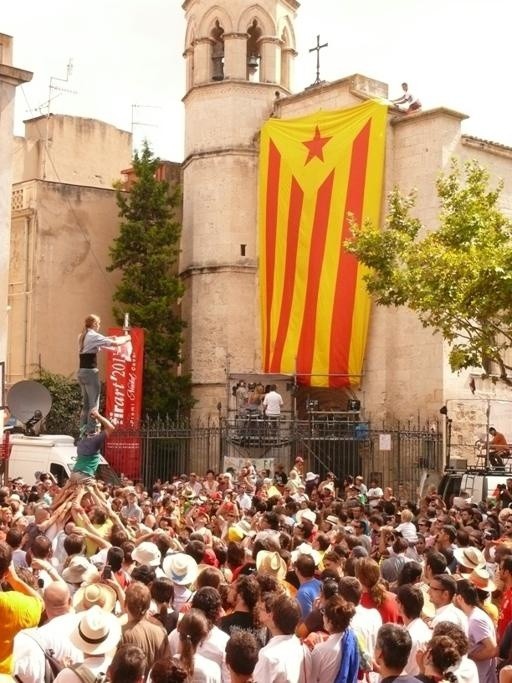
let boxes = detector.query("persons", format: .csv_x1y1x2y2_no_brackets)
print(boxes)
272,91,283,102
75,312,132,438
0,455,512,683
69,410,115,484
387,82,424,113
479,426,509,472
232,378,284,436
3,406,26,428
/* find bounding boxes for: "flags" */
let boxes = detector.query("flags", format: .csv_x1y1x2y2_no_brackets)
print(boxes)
258,98,389,383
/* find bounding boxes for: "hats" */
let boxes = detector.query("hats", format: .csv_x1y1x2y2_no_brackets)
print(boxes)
453,547,486,568
256,550,287,580
263,478,273,484
460,569,497,592
125,488,136,496
182,487,195,498
7,494,21,503
296,457,304,462
61,555,122,656
305,472,319,482
325,515,338,526
131,542,199,585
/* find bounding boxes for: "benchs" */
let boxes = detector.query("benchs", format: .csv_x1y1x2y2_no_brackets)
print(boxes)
475,454,512,472
